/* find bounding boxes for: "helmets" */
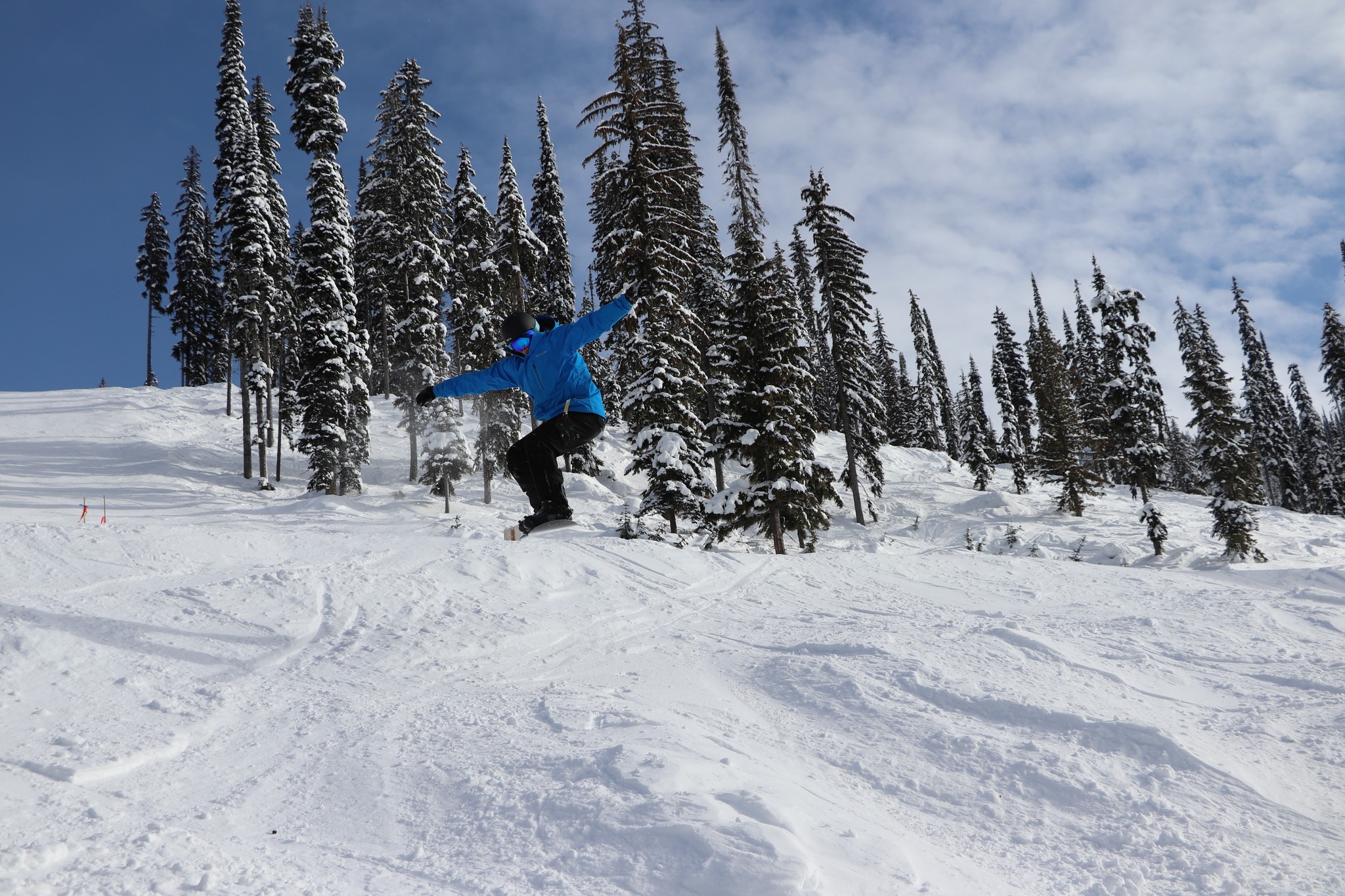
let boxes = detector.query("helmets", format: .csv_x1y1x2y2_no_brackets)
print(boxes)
501,312,536,345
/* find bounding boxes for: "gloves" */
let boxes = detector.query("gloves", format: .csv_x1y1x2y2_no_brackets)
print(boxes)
416,386,436,408
625,280,655,304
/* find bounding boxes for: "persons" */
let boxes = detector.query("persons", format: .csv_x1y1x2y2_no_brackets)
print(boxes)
416,279,655,531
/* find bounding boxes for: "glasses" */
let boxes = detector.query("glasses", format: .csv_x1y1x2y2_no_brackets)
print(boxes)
511,330,534,352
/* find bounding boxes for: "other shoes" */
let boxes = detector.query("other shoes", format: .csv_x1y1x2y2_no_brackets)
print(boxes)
525,507,573,530
518,510,540,533
